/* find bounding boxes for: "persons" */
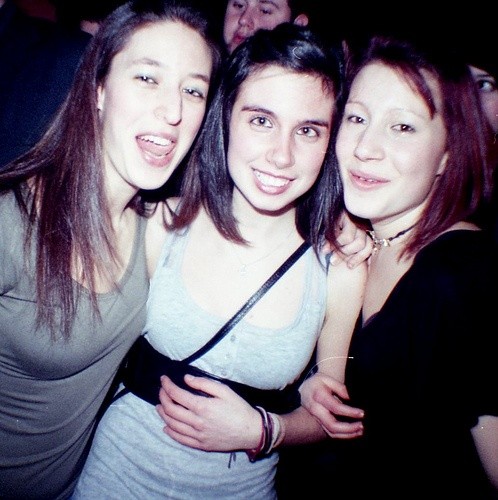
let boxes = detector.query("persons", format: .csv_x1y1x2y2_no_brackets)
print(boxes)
1,0,375,500
20,1,113,141
71,27,368,500
279,30,498,500
223,1,309,56
470,65,498,135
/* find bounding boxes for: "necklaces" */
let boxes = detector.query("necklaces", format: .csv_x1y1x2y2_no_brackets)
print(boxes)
368,217,423,251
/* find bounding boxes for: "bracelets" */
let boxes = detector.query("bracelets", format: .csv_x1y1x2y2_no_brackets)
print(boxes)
245,405,285,465
